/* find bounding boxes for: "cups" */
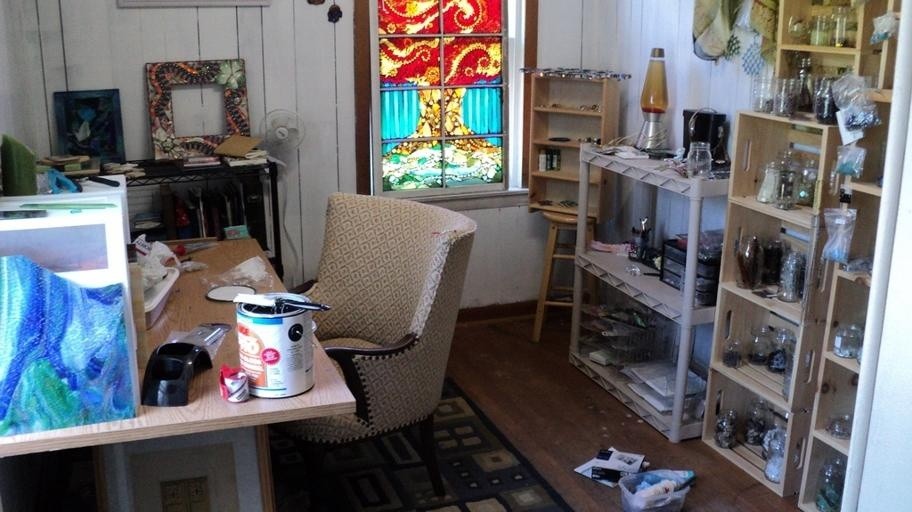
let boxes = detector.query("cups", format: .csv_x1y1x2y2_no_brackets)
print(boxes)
754,77,774,113
774,78,802,117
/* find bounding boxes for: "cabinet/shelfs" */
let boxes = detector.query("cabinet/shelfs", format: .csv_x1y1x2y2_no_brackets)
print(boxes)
568,145,734,444
772,1,902,129
798,177,889,512
41,157,285,286
701,111,886,498
528,73,623,223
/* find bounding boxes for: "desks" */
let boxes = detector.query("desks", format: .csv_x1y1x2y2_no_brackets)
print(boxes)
0,238,356,511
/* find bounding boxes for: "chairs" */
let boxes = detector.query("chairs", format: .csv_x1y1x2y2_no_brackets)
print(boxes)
258,190,478,512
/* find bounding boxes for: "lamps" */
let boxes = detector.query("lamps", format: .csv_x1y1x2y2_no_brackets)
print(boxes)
633,47,670,151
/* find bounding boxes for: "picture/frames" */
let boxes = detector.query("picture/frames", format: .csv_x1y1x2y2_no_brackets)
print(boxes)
148,59,251,161
55,88,123,166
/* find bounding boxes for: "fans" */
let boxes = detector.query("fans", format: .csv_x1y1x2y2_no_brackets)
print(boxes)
260,110,304,158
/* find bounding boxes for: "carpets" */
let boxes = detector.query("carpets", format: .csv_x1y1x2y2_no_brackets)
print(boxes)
268,372,572,511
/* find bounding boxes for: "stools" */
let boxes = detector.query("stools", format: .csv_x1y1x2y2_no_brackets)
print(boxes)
532,211,598,344
